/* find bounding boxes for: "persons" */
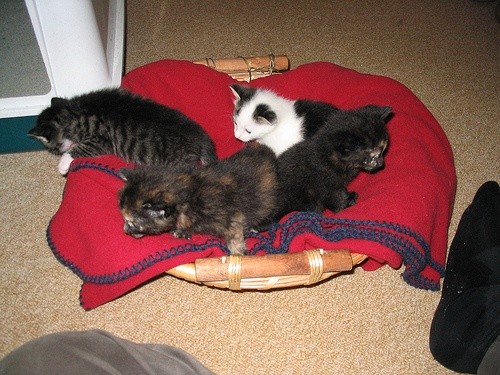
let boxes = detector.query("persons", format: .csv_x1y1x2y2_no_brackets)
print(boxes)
0,181,500,375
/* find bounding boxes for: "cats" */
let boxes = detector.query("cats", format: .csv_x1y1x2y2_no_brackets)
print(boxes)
228,84,344,159
28,87,218,175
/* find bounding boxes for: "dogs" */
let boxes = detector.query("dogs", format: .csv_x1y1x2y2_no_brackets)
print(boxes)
115,104,392,256
